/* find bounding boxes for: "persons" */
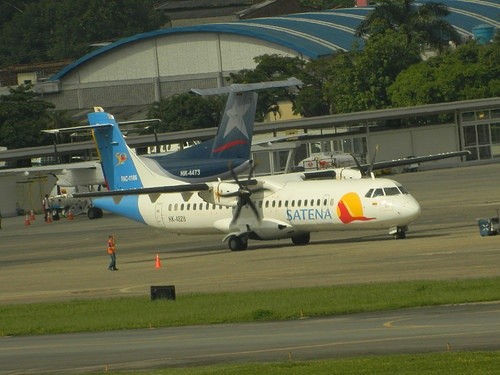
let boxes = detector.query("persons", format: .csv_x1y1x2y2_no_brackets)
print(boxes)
14,194,62,223
105,234,119,271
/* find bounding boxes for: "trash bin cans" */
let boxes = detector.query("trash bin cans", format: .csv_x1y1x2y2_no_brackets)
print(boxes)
478,217,498,236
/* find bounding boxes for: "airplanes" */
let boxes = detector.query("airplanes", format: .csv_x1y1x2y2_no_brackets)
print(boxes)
41,76,421,252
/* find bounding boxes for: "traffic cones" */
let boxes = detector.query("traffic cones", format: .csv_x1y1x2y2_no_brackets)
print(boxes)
67,208,74,220
24,214,30,225
46,211,52,223
30,209,35,220
154,254,161,269
